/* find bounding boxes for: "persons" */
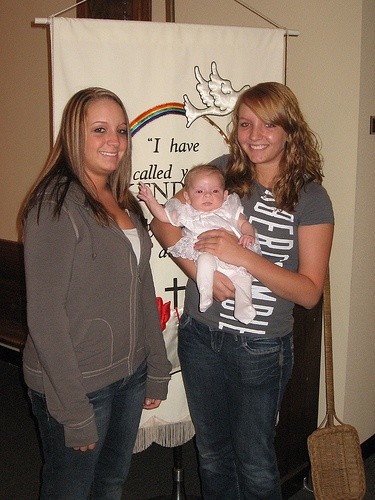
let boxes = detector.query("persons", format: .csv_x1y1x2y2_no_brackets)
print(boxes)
137,165,262,324
18,87,173,500
149,82,335,500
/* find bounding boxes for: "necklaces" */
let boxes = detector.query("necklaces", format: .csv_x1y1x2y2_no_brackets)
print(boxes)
256,175,272,198
